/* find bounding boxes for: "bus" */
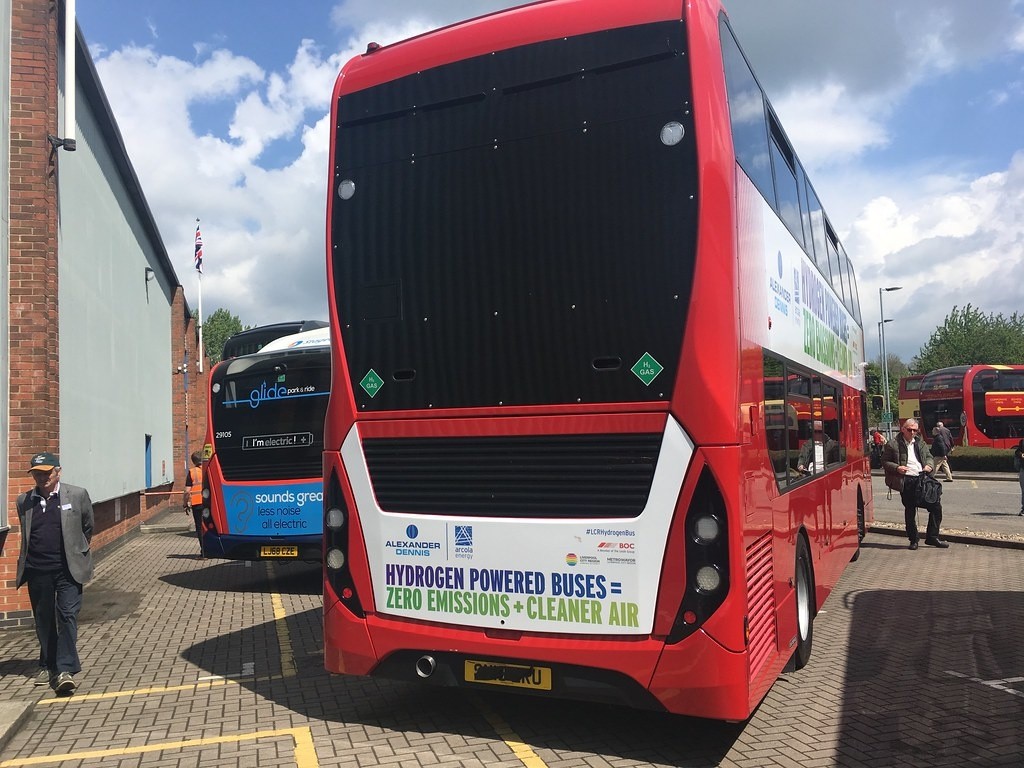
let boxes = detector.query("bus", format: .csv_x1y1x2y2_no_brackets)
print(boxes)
900,376,997,443
919,363,1024,450
222,321,330,361
322,0,884,724
199,325,332,565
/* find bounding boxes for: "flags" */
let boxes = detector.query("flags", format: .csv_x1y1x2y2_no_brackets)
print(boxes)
195,224,203,274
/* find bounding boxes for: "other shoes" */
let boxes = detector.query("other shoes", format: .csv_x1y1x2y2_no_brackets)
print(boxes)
943,479,953,482
194,553,207,560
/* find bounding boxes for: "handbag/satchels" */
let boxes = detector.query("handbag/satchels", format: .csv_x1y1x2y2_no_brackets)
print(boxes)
915,471,942,509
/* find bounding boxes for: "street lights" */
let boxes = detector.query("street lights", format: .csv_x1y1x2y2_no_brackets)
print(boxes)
175,364,189,478
879,286,902,440
878,319,893,439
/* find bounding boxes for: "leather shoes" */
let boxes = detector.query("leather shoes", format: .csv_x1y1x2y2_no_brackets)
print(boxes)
925,537,949,548
909,541,918,550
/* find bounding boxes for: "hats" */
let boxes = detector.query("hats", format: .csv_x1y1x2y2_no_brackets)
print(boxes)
27,453,60,473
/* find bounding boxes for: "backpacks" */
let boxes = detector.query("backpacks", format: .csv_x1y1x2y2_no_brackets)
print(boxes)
876,431,887,446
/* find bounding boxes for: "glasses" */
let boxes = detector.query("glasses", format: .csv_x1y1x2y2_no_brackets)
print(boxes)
904,427,918,432
814,430,823,434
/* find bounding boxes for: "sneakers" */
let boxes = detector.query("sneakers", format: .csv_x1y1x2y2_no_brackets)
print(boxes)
58,672,76,690
34,669,59,685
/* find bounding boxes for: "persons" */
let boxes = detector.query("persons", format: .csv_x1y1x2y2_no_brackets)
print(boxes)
1015,429,1024,516
881,418,949,550
1012,380,1019,391
928,422,955,482
16,453,94,693
973,379,984,391
1009,423,1024,438
797,421,834,477
870,429,887,469
182,450,208,560
993,378,1000,391
